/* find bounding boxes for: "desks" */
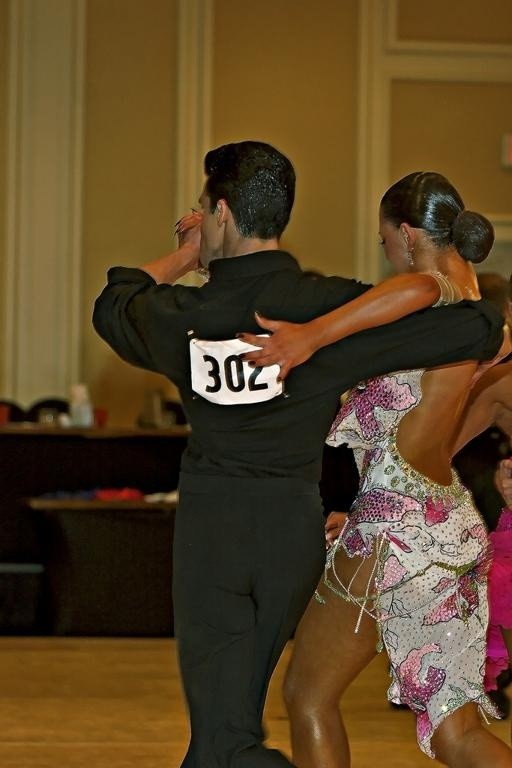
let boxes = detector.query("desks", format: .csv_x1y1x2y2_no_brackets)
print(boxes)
3,423,359,638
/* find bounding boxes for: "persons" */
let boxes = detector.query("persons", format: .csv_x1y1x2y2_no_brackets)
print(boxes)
482,458,512,692
237,171,512,768
91,140,505,768
